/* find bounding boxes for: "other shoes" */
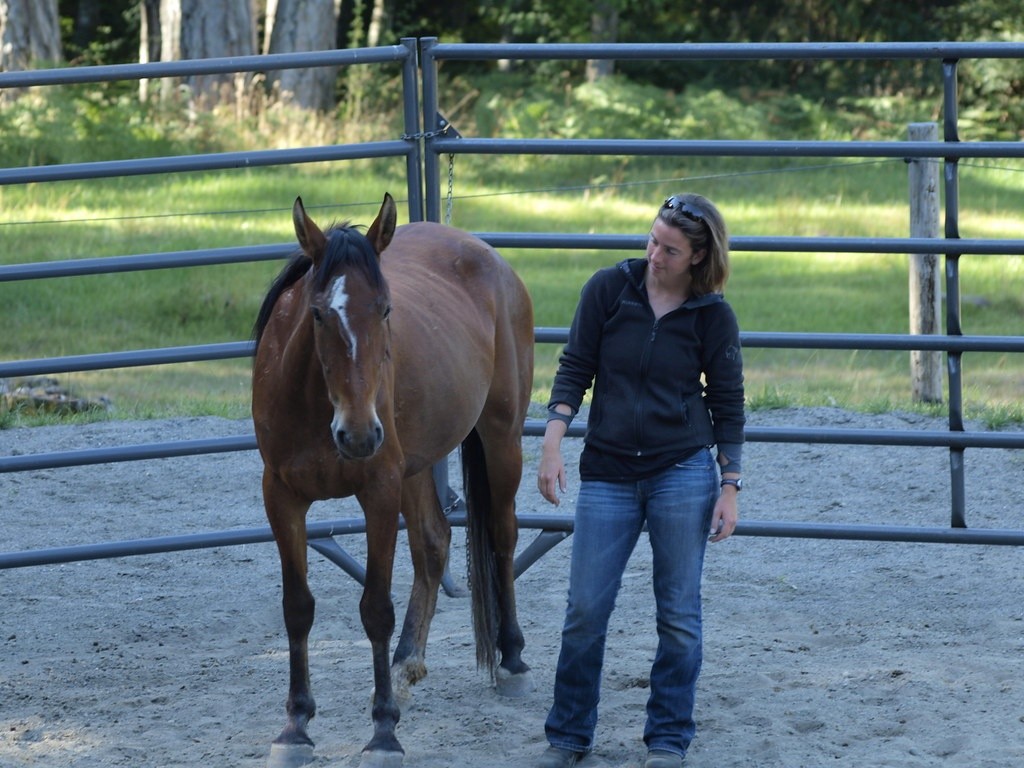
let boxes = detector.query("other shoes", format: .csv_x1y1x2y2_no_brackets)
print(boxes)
646,748,686,768
537,745,590,768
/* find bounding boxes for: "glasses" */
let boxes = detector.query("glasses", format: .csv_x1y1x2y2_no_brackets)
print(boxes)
665,196,704,222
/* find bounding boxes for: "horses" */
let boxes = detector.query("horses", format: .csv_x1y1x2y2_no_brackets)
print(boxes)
245,190,536,767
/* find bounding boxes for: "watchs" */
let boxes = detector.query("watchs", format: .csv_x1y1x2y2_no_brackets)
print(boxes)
719,477,743,492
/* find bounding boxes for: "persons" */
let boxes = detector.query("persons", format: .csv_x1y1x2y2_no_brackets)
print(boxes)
535,189,746,768
